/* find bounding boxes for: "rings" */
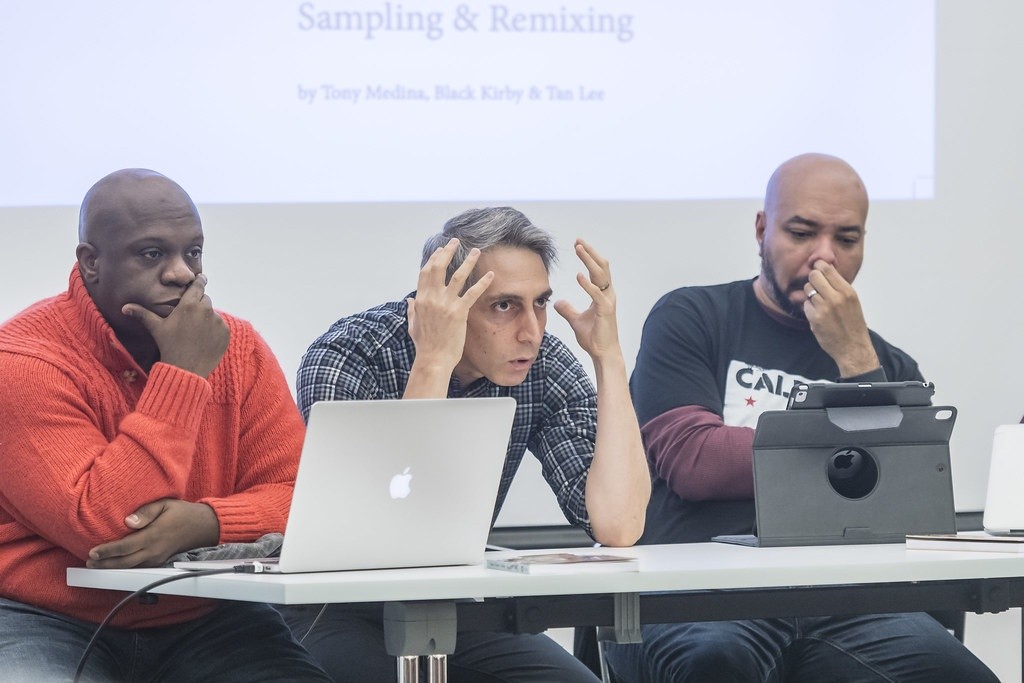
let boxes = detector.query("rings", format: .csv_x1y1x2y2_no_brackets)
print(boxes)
599,281,610,291
806,289,817,298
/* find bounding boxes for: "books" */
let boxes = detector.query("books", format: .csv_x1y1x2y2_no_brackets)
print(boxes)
905,534,1024,554
486,553,640,576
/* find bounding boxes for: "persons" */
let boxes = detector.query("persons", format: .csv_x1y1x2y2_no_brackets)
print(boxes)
0,167,338,683
269,206,652,683
597,152,1002,683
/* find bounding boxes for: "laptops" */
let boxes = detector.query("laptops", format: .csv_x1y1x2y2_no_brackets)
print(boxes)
172,396,518,575
981,424,1024,539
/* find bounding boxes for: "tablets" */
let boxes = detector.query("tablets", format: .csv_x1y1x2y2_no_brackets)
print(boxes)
786,380,935,412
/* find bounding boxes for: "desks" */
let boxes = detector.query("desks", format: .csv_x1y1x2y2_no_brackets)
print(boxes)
66,529,1024,683
